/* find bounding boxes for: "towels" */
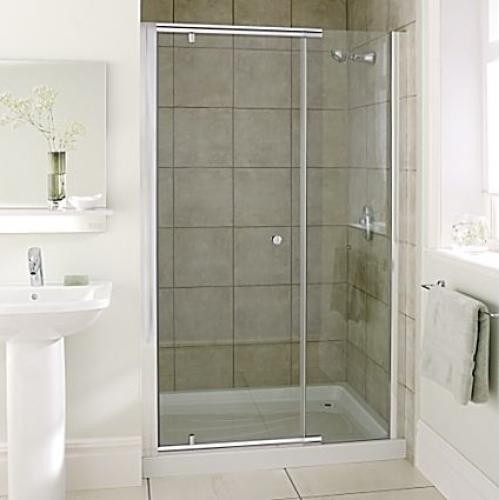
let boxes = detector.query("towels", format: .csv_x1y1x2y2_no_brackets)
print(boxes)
420,287,491,406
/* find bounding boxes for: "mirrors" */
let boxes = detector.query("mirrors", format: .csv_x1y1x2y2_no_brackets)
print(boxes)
1,59,107,209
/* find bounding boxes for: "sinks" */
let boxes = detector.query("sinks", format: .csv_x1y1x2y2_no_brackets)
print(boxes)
0,279,112,339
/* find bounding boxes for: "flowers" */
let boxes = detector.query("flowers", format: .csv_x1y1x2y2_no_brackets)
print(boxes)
2,85,84,152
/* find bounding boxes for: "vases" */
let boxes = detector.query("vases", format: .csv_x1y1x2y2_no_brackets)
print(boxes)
47,153,65,203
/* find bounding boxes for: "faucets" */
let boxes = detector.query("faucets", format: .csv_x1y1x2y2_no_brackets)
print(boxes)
27,246,42,276
